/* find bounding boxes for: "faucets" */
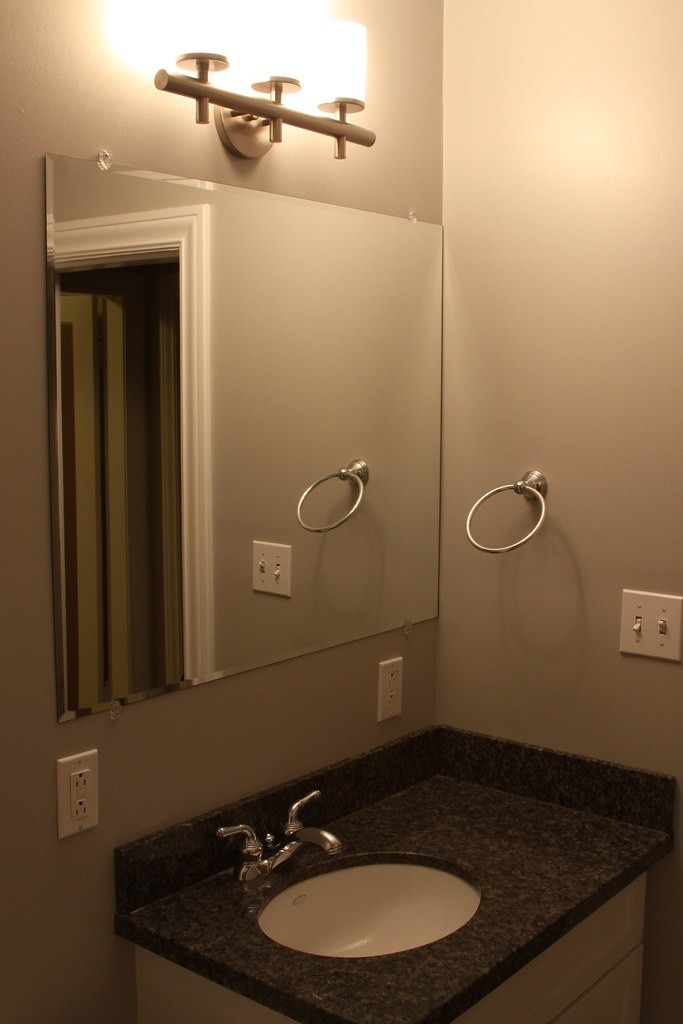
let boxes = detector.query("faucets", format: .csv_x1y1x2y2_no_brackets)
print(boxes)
274,826,348,871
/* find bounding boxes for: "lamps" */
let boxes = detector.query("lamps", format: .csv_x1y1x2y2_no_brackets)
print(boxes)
154,0,376,159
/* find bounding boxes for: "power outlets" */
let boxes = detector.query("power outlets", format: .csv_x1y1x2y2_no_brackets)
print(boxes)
378,657,403,722
56,749,99,839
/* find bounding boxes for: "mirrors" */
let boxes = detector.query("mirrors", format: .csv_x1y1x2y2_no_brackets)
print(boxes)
45,153,443,726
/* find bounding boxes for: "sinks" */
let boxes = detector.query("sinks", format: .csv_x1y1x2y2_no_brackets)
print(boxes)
256,851,485,957
